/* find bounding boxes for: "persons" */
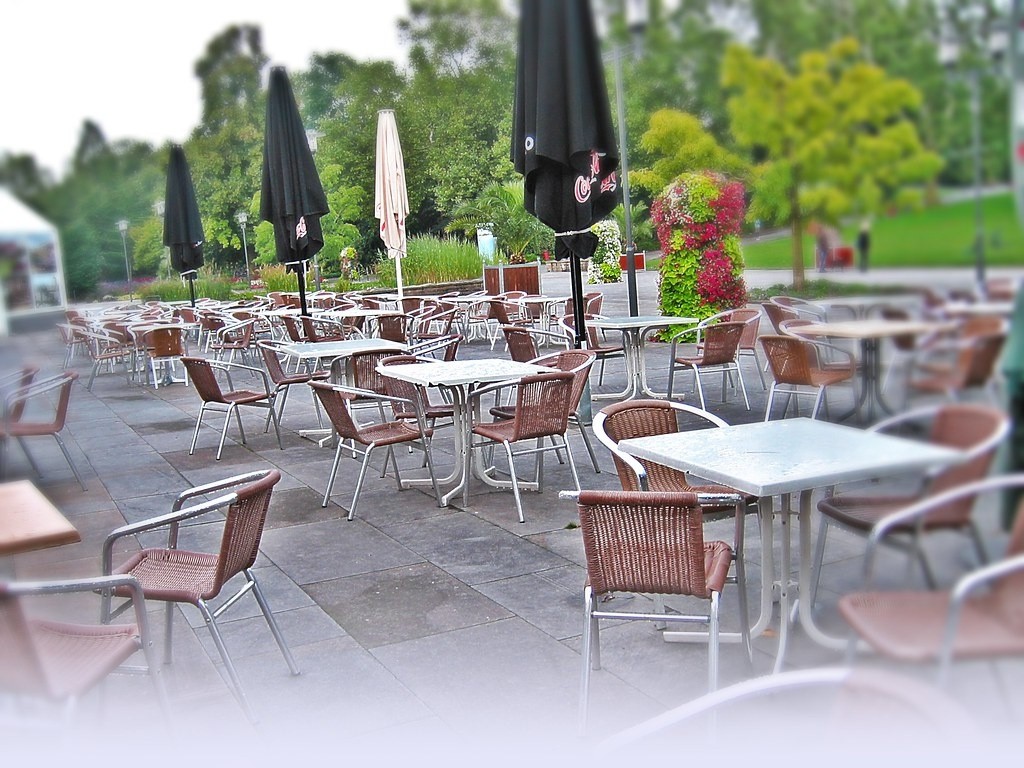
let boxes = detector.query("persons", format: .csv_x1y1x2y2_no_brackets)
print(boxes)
754,211,875,274
340,255,373,283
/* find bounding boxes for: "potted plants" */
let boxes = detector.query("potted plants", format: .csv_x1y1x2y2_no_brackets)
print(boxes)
446,180,554,297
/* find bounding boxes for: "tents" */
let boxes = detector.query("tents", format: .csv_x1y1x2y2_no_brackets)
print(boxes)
0,186,70,316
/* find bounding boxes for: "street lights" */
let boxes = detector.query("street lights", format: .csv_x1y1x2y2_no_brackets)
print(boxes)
114,221,133,303
938,23,1010,303
152,202,172,280
304,129,326,291
594,0,649,318
235,213,251,289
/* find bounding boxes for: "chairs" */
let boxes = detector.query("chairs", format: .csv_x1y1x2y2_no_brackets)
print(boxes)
0,289,1023,768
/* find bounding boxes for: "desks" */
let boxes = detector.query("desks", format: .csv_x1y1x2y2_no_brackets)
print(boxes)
442,296,506,343
316,309,400,339
788,320,942,428
0,480,81,582
572,315,698,402
280,338,407,448
617,417,969,680
84,315,126,320
509,297,569,349
132,322,197,385
253,307,324,348
375,358,561,508
808,295,924,372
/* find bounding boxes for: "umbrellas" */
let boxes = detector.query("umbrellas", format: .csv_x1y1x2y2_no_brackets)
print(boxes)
256,64,332,341
373,106,413,313
162,141,205,321
509,0,624,425
